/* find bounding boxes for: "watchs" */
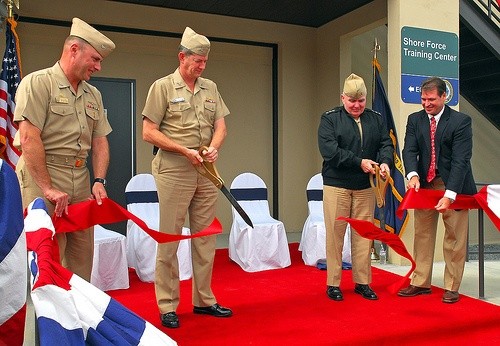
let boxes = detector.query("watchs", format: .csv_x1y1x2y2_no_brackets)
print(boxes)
93,178,106,187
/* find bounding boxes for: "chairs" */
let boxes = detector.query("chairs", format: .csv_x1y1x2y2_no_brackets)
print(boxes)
125,173,193,282
90,224,130,291
298,172,352,269
229,172,291,273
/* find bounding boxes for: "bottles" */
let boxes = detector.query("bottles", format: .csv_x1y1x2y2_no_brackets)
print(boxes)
380,244,386,264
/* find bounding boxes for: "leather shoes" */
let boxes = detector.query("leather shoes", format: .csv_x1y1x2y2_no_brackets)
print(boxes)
327,286,342,300
354,283,377,299
398,285,432,296
194,303,232,317
443,291,460,302
161,311,179,327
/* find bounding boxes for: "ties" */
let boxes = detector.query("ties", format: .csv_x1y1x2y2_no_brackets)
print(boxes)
426,117,436,183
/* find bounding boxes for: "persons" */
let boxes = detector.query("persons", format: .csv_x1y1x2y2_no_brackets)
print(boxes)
142,26,232,326
14,16,116,282
317,74,393,301
397,78,476,303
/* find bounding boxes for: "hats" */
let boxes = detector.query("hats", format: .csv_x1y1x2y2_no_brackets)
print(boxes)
343,72,367,98
180,26,211,56
71,17,116,60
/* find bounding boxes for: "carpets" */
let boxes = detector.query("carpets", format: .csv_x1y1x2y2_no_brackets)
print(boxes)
103,242,500,346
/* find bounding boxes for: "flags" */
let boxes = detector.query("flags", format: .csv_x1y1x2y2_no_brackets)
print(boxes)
372,58,408,237
0,17,23,174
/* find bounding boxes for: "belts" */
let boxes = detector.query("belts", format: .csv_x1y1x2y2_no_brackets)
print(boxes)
45,154,87,166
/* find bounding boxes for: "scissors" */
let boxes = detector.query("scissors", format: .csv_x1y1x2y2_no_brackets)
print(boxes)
191,146,255,229
369,163,391,251
